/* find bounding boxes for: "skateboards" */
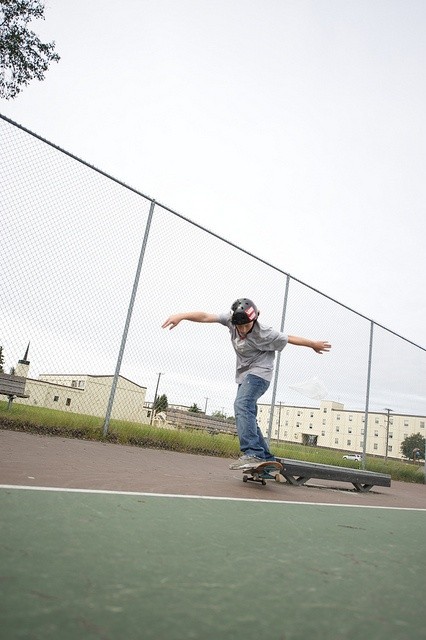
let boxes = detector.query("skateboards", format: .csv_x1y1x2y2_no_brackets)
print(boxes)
226,461,283,485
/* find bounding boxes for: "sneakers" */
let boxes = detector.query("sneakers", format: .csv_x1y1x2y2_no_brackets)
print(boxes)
229,455,267,469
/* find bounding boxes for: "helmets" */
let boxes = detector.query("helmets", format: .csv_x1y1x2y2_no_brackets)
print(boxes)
229,298,258,325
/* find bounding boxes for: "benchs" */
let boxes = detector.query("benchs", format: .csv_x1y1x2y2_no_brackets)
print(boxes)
274,457,391,493
166,407,238,439
0,373,30,411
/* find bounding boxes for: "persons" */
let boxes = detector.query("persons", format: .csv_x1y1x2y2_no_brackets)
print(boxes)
161,299,332,469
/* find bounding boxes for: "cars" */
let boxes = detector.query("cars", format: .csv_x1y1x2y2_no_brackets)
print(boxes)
343,454,362,461
